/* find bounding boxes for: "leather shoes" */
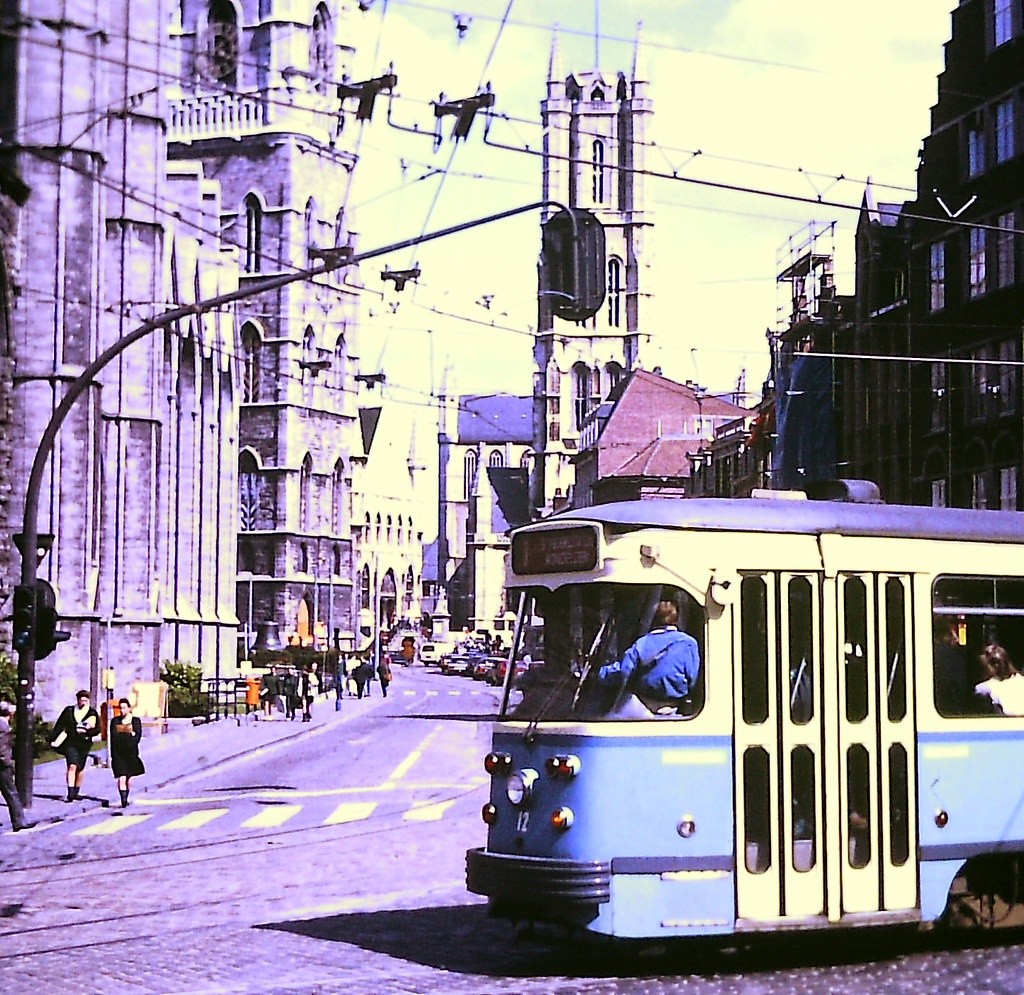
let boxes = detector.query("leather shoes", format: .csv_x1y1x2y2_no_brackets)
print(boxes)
13,821,38,832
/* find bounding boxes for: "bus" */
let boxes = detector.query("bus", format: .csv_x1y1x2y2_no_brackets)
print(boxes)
466,496,1024,952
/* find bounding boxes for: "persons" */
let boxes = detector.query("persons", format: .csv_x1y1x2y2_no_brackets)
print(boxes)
282,667,300,723
259,666,283,722
296,664,320,723
377,659,393,699
336,651,375,700
109,698,145,808
48,690,103,803
742,582,875,843
971,645,1024,720
0,701,40,833
934,613,988,717
307,661,324,719
581,599,702,718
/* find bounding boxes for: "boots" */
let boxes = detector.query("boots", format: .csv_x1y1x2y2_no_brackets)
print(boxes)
119,790,130,807
73,786,83,800
67,787,75,801
